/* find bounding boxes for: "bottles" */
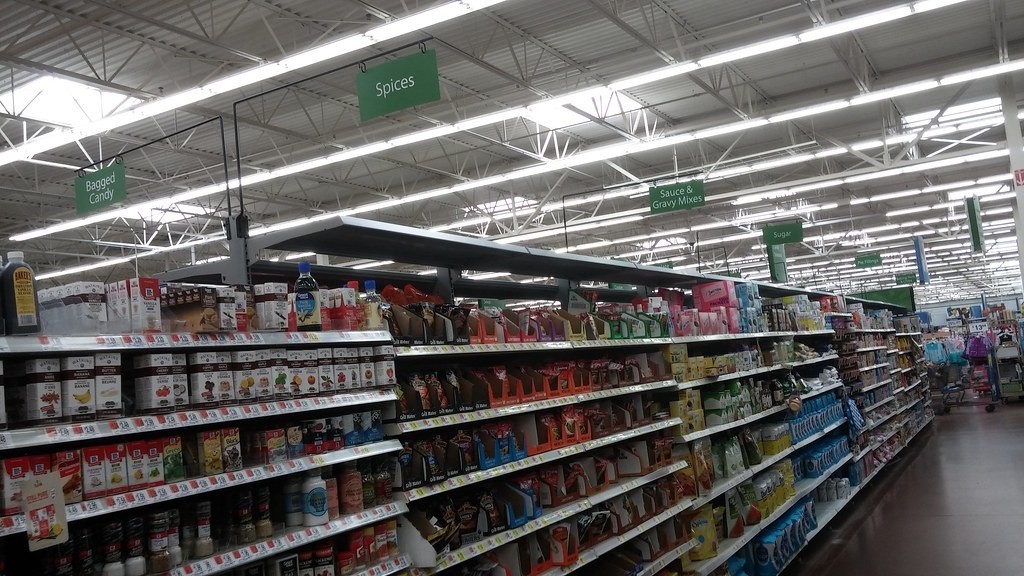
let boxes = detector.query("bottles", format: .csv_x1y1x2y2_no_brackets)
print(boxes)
40,516,49,537
294,261,323,332
0,252,41,336
342,280,382,330
31,516,40,537
0,458,397,576
49,511,57,528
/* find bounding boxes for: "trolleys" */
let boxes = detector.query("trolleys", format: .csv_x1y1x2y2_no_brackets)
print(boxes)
990,319,1024,405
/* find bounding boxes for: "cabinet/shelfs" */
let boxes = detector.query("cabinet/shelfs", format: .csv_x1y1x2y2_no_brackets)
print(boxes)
0,307,1024,576
